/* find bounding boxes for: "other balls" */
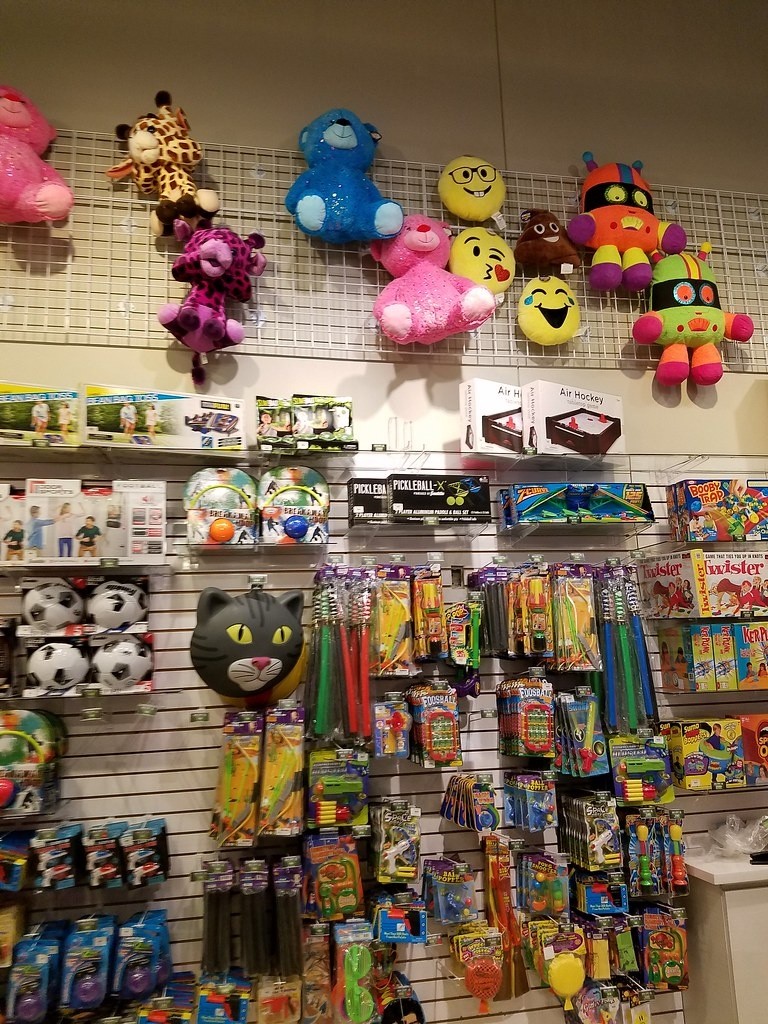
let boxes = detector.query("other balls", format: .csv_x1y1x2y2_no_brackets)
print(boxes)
285,515,309,538
0,779,17,809
210,517,234,541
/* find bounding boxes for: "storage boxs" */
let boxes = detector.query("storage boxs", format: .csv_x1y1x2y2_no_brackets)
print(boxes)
457,377,551,469
709,624,738,692
81,382,247,450
386,474,492,530
0,573,156,700
658,717,722,787
703,552,768,617
495,488,635,536
666,484,685,544
347,478,453,530
725,713,768,786
657,624,717,693
675,478,768,542
670,718,747,789
0,484,34,577
23,477,171,577
633,548,712,621
256,396,312,457
0,380,81,448
291,394,358,454
731,621,768,691
507,482,655,530
521,380,625,470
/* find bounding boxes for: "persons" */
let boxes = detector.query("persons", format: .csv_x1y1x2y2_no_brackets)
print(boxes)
144,405,158,435
58,502,87,557
3,520,24,560
31,402,51,432
120,403,137,438
75,516,106,557
26,506,72,556
57,402,75,431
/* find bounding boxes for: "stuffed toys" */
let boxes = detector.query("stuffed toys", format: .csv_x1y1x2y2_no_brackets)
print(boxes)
285,108,404,245
371,151,754,386
106,90,220,239
158,219,267,385
0,86,72,224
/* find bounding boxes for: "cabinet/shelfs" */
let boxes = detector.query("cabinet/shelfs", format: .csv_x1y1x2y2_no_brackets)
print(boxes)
682,846,768,1024
0,440,768,703
